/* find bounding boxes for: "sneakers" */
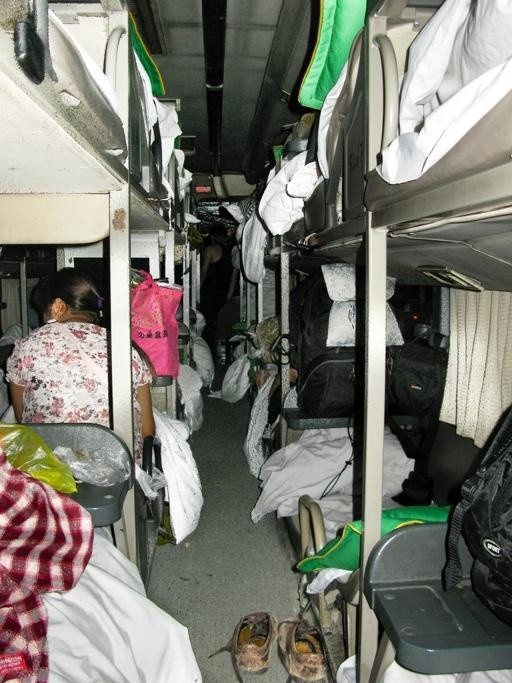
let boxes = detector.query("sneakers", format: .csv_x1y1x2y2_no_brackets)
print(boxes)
230,611,277,682
277,617,329,683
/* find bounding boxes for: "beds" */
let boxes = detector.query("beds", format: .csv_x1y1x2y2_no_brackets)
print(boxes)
239,0,512,681
0,0,200,682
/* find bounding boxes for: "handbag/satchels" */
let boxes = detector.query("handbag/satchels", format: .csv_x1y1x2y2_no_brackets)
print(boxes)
132,281,185,378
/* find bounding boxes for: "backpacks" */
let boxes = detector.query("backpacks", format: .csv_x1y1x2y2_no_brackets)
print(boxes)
461,439,512,625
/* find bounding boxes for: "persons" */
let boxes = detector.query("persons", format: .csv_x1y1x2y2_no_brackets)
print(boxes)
2,259,153,465
197,220,239,366
